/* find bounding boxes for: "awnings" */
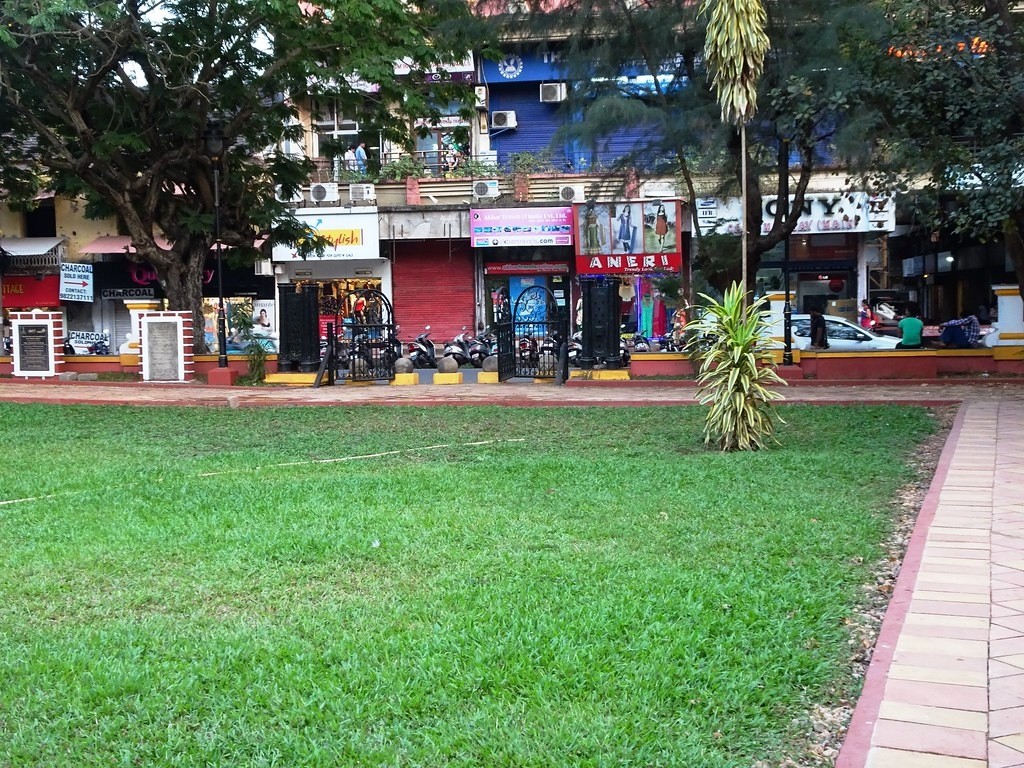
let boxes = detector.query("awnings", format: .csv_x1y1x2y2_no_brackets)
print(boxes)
0,237,66,256
78,235,271,255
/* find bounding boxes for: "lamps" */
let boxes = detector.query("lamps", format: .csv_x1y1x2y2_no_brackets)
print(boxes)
295,269,312,278
354,269,373,277
36,270,46,280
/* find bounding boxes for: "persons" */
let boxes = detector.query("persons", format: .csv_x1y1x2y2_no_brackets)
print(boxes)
353,292,381,343
859,299,872,330
930,311,980,349
256,309,271,331
810,305,828,350
568,295,584,332
344,140,368,175
654,205,668,253
585,208,603,255
895,301,924,349
617,205,637,252
756,290,771,318
443,144,465,178
989,306,998,326
791,304,797,314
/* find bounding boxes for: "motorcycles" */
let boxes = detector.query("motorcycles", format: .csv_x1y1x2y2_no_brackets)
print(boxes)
92,328,114,355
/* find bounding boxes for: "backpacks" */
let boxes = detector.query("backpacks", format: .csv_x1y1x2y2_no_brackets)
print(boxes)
871,312,880,328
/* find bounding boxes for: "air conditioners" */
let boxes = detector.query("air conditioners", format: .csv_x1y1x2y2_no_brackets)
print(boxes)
274,183,303,203
473,179,499,198
559,185,584,202
492,111,518,129
540,83,568,103
349,183,376,201
310,182,338,201
474,86,489,111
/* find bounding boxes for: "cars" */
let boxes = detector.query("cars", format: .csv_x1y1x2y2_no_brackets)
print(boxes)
226,332,277,354
751,313,902,351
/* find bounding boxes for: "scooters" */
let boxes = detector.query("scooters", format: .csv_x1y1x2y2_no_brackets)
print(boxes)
619,323,680,367
407,325,438,369
320,324,403,369
442,324,582,368
62,337,75,357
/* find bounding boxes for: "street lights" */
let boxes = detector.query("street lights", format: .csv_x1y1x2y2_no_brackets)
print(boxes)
771,107,799,364
198,106,230,365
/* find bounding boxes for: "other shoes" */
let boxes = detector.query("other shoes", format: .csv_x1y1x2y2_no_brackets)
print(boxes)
931,340,946,348
659,239,661,244
623,251,627,254
626,249,632,254
660,249,663,253
